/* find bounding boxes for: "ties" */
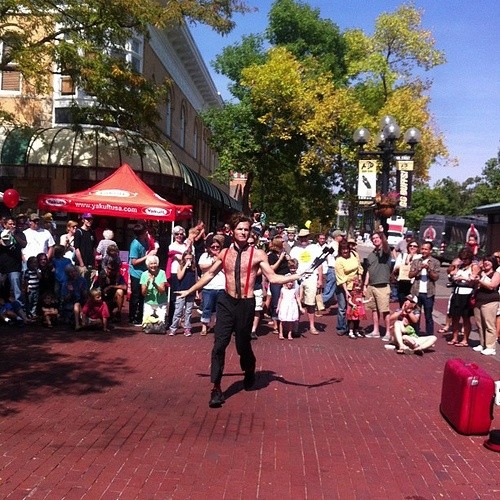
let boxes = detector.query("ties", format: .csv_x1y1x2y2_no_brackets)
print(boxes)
234,242,248,299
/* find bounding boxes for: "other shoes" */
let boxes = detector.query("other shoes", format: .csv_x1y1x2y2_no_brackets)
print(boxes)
200,330,207,336
396,349,424,356
438,328,469,347
244,370,255,389
337,330,363,339
111,317,142,327
271,328,319,340
210,389,224,408
169,330,192,336
16,317,81,330
251,332,257,339
197,309,203,314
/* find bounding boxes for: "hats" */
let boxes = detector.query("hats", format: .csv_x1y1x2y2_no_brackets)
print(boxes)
29,213,40,220
82,212,94,218
483,430,500,452
333,230,357,245
298,229,310,236
405,294,418,303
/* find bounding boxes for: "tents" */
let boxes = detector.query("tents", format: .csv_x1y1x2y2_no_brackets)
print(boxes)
35,163,197,303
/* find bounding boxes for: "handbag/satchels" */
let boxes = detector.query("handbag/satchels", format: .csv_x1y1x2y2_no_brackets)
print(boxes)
143,321,166,334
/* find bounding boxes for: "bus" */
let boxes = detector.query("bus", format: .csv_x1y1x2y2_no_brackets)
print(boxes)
419,214,489,265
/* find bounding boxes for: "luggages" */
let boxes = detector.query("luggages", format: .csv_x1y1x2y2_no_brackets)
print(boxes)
440,357,496,436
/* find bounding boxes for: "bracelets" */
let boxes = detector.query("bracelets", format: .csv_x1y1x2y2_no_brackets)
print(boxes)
79,264,85,267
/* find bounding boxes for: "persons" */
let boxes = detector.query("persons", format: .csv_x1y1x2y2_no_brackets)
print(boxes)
73,287,113,336
408,241,441,336
289,228,324,334
168,251,197,336
198,238,226,335
172,214,303,407
0,216,27,322
0,204,500,357
473,254,500,357
41,290,64,329
21,212,56,272
139,254,167,326
362,224,392,342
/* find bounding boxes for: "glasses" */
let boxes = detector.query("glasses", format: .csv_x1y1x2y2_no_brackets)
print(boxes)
211,246,219,248
409,245,418,248
175,232,183,235
30,220,39,222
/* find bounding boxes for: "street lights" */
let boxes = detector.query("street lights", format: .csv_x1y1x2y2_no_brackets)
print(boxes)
354,116,422,240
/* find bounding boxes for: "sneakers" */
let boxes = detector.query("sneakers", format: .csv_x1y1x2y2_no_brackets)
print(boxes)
365,332,390,341
472,345,497,355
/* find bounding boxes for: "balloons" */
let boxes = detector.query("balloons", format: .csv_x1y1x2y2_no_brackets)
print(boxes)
3,189,20,209
